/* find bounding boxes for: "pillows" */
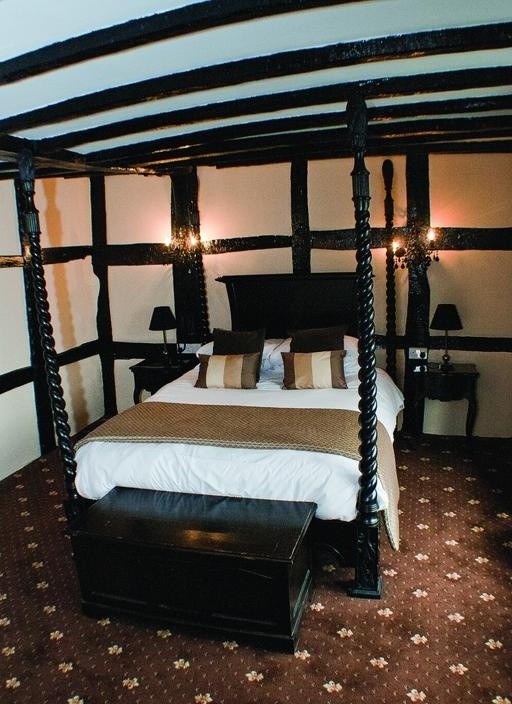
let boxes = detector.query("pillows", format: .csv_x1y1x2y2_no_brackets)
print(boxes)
194,320,361,389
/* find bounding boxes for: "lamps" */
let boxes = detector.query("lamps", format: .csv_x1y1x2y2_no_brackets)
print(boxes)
429,301,464,370
147,305,179,362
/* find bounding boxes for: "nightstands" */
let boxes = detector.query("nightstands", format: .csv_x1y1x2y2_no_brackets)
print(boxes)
128,351,200,405
408,360,480,456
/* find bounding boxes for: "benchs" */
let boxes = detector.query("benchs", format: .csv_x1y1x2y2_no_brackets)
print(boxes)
66,484,317,657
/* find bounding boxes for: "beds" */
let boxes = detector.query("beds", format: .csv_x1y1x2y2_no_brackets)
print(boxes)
60,271,403,571
